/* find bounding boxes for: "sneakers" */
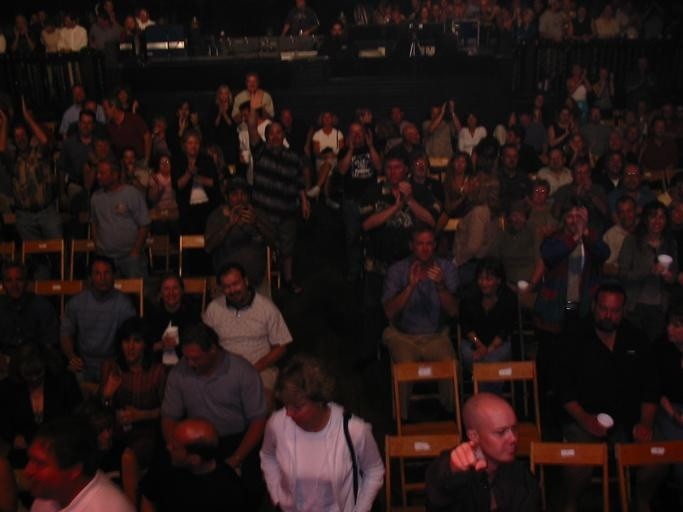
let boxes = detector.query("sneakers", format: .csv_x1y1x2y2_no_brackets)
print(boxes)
308,186,339,208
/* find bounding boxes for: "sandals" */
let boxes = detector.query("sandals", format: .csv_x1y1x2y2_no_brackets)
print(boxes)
286,279,303,294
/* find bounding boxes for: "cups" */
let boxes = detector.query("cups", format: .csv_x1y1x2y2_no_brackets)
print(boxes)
166,326,179,345
595,413,614,430
658,254,673,275
517,280,529,291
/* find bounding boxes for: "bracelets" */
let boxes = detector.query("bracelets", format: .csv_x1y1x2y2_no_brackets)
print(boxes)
232,454,242,462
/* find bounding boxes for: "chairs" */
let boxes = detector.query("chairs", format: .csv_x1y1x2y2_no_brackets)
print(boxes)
383,360,464,510
616,440,682,512
443,217,462,234
0,230,280,322
528,443,610,511
428,152,450,181
470,361,543,502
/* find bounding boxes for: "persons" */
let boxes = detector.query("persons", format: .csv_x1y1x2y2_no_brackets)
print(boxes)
427,392,544,511
258,376,386,512
557,283,659,512
135,418,249,510
157,322,274,482
0,339,82,512
23,417,135,512
636,296,683,512
97,316,170,511
2,0,683,407
